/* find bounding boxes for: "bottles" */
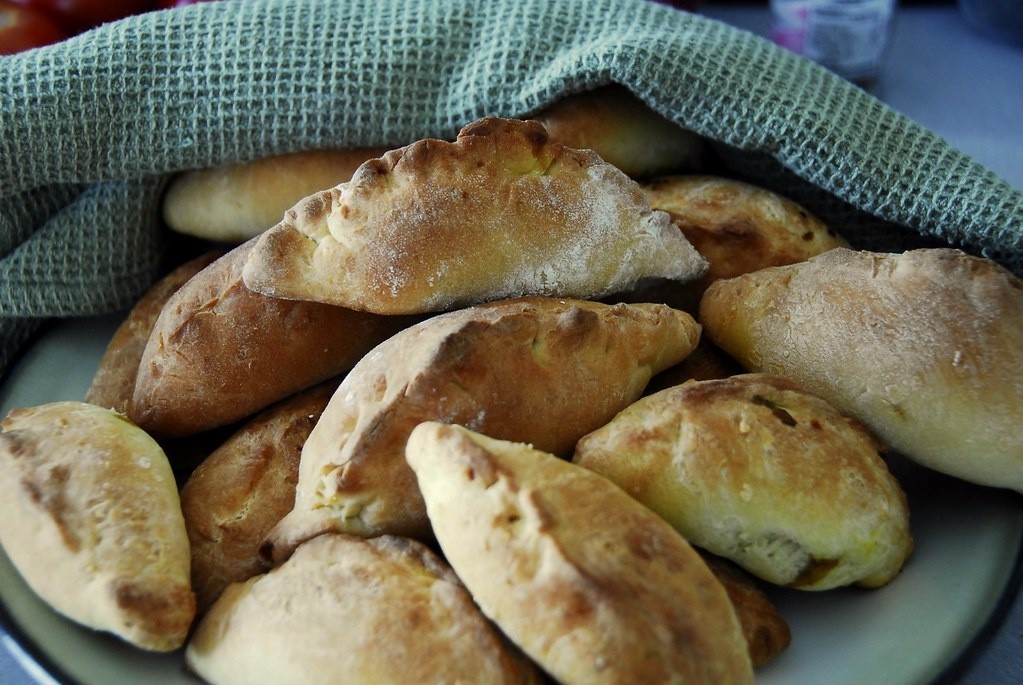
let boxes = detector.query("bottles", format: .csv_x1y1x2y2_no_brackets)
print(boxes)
771,0,894,85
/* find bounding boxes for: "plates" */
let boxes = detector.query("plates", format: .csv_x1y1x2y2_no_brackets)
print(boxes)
0,321,1023,685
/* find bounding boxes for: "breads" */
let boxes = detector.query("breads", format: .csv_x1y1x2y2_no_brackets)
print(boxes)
0,82,1022,685
